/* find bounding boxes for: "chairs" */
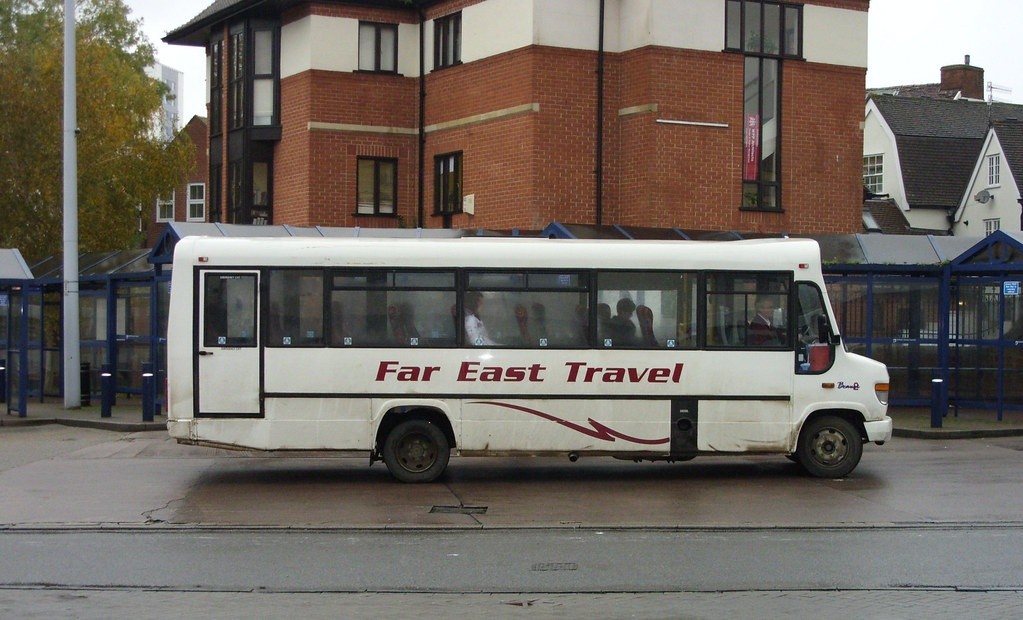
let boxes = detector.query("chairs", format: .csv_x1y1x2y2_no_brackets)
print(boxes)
739,321,750,350
204,303,661,350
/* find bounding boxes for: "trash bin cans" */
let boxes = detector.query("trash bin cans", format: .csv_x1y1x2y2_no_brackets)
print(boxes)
79,359,93,408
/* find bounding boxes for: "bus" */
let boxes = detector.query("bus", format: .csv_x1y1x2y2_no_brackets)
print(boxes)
165,236,895,482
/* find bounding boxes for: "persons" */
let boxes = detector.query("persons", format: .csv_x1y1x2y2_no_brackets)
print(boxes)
609,298,638,348
745,295,804,347
463,292,499,348
